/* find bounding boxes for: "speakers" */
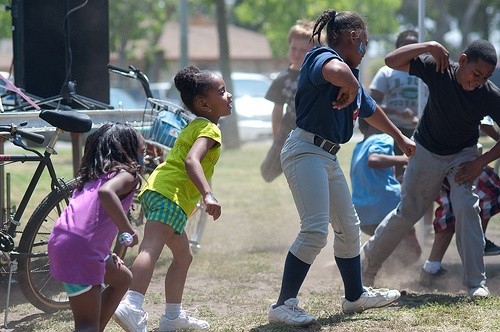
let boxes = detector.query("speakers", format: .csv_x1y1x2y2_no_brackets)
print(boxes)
10,0,110,107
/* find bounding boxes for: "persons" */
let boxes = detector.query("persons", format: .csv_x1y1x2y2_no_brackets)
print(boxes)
419,118,500,280
361,38,500,300
260,18,326,183
111,64,233,332
268,10,417,327
48,121,149,332
349,101,422,268
369,29,430,184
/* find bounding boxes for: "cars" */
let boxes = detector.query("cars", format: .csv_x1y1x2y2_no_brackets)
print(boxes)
147,81,183,105
109,88,139,110
228,72,287,144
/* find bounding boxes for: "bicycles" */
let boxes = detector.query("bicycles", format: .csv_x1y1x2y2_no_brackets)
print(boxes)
106,62,226,253
0,109,132,329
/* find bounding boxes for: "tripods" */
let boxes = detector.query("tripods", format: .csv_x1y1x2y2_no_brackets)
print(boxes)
4,0,118,112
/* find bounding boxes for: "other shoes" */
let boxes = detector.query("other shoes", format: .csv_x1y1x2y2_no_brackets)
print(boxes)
483,237,500,254
420,267,448,280
469,286,490,298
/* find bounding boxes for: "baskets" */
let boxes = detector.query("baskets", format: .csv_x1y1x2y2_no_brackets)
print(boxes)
141,97,193,170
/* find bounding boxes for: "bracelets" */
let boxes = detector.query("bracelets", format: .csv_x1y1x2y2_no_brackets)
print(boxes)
204,191,212,196
119,230,137,246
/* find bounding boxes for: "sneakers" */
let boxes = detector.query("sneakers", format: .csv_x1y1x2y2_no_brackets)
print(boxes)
112,299,148,332
158,310,210,332
341,286,400,314
268,298,317,326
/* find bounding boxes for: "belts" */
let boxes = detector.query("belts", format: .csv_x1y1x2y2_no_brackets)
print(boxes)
314,135,340,155
